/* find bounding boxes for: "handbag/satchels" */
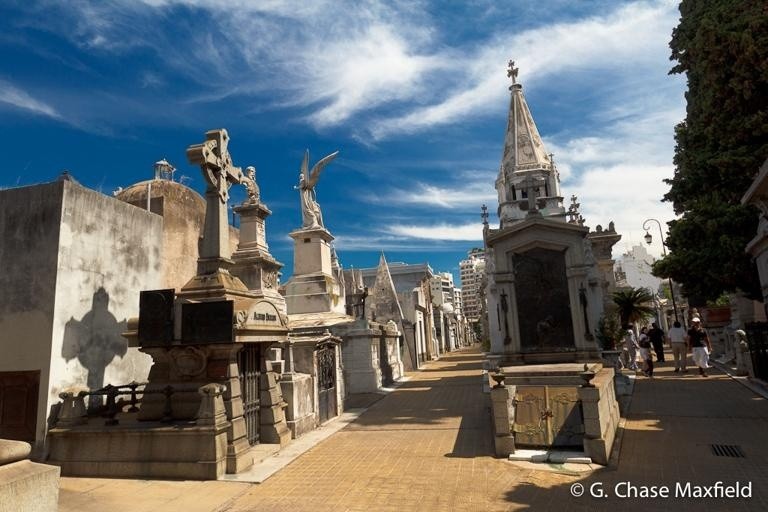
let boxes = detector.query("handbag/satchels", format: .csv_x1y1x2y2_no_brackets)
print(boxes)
639,337,651,348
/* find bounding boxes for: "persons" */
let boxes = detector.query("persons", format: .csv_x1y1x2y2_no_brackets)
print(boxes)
679,308,685,321
734,329,748,353
637,326,651,372
624,323,639,371
666,321,688,373
645,342,656,377
646,323,666,363
685,316,712,378
690,307,700,318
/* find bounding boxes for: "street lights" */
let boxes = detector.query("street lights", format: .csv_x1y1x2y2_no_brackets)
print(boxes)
642,218,679,325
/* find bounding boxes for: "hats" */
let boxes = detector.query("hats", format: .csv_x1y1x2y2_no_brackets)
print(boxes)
691,317,700,322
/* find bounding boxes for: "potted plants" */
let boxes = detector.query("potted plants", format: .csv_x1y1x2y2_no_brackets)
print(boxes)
699,295,731,322
594,312,616,351
